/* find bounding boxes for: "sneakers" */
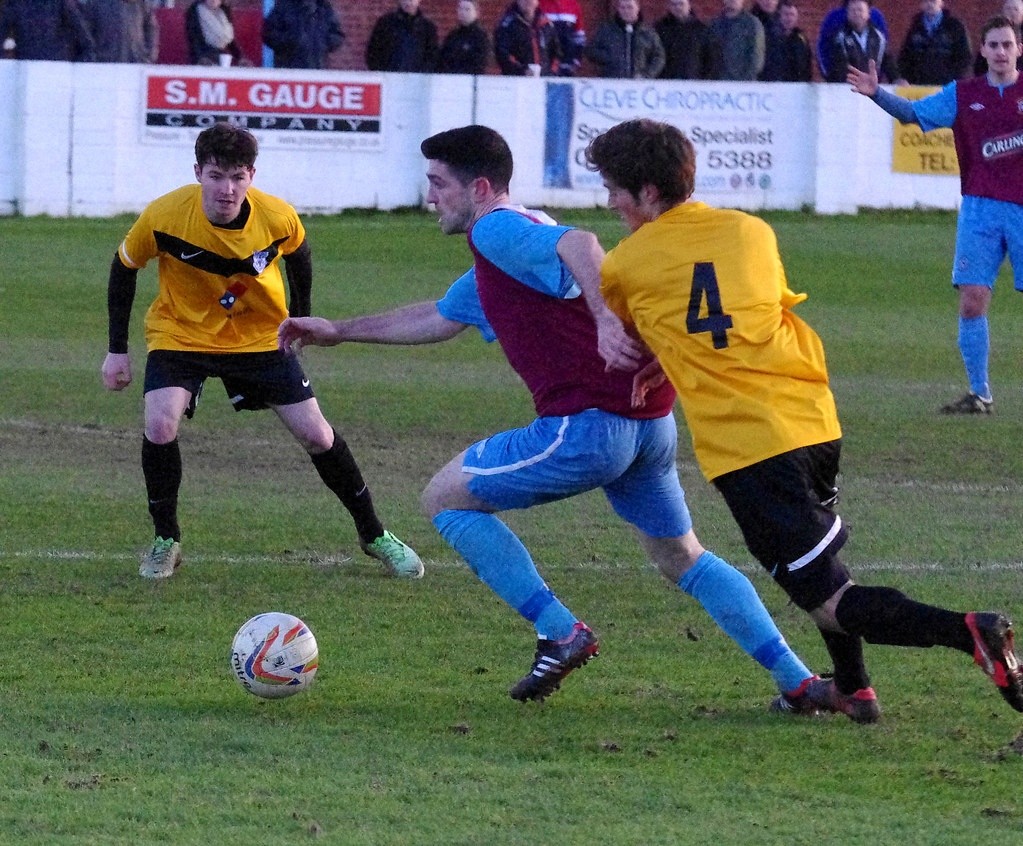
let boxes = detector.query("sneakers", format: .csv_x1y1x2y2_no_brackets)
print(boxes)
359,531,425,582
138,536,185,578
806,678,883,726
510,620,599,701
768,675,833,717
938,390,995,417
965,610,1023,714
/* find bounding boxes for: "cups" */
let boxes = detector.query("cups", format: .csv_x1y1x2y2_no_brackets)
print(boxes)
529,64,542,77
4,37,16,60
219,53,234,66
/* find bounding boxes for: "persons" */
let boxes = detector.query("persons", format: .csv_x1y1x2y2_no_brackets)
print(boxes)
654,0,813,82
186,0,241,66
592,119,1023,723
816,0,970,84
493,0,665,78
0,0,158,65
262,0,346,68
847,17,1023,413
364,0,490,75
278,125,828,710
974,0,1023,76
102,123,425,580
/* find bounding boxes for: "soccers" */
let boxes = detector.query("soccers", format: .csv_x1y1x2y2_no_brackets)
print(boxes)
230,612,319,697
